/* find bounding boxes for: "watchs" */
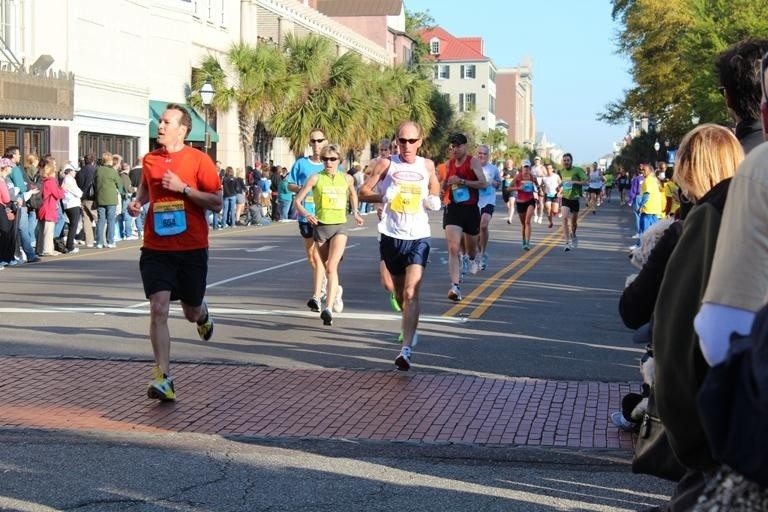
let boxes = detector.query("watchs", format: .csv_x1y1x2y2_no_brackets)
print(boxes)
182,183,193,195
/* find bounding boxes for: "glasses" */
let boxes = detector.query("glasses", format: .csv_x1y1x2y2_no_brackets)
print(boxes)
321,156,339,162
309,138,325,144
397,138,419,145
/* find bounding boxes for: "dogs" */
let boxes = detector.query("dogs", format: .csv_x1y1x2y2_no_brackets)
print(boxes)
624,215,680,388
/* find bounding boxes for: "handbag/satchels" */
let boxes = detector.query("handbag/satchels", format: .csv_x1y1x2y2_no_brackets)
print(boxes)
629,406,682,482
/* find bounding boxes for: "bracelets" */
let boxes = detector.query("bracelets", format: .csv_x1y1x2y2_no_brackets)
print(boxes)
351,208,359,214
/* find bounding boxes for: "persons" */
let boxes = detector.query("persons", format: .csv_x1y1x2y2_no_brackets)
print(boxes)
380,134,405,341
293,145,364,326
127,104,223,402
287,128,342,312
439,133,487,301
358,121,441,371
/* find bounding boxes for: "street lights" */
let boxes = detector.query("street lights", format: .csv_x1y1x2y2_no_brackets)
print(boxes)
653,139,661,166
663,137,670,162
690,109,701,127
199,74,215,155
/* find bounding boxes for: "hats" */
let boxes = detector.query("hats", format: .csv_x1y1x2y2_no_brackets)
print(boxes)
449,135,467,144
520,158,531,167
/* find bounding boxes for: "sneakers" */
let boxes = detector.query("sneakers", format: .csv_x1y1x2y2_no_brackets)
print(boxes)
521,242,531,252
506,213,564,228
334,286,345,314
563,236,580,254
308,296,320,313
196,302,215,343
447,285,464,302
459,253,469,273
469,259,479,275
322,309,333,326
389,289,402,314
393,347,414,372
0,232,144,268
611,410,640,433
397,333,405,341
479,254,488,271
146,373,178,404
